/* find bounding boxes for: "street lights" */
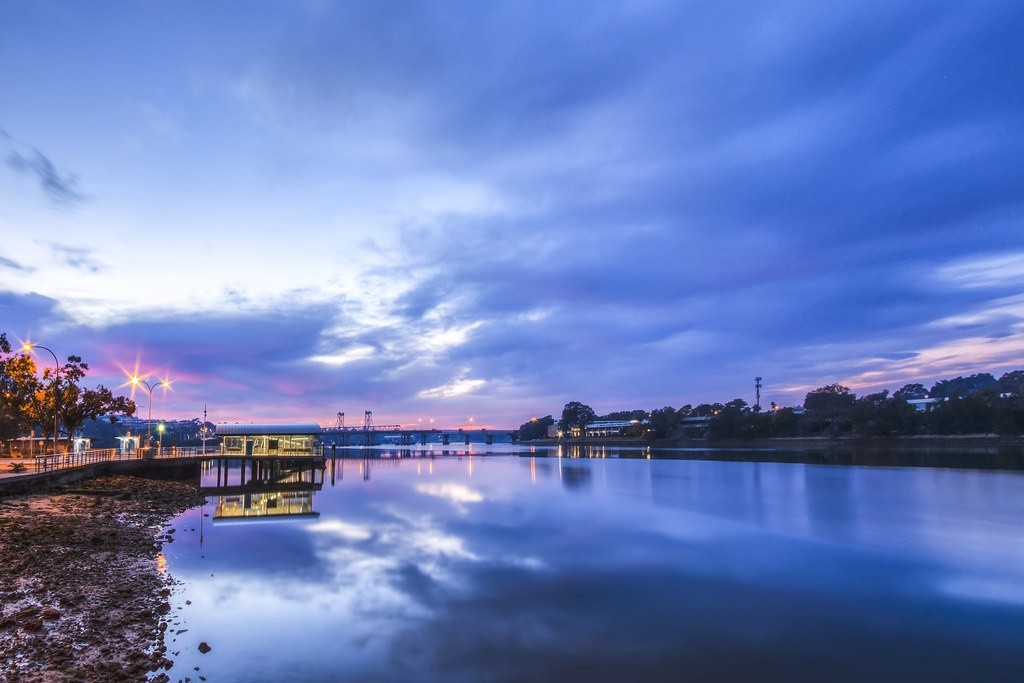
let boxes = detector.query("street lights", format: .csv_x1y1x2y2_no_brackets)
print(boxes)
25,344,59,466
132,378,168,448
158,424,164,456
